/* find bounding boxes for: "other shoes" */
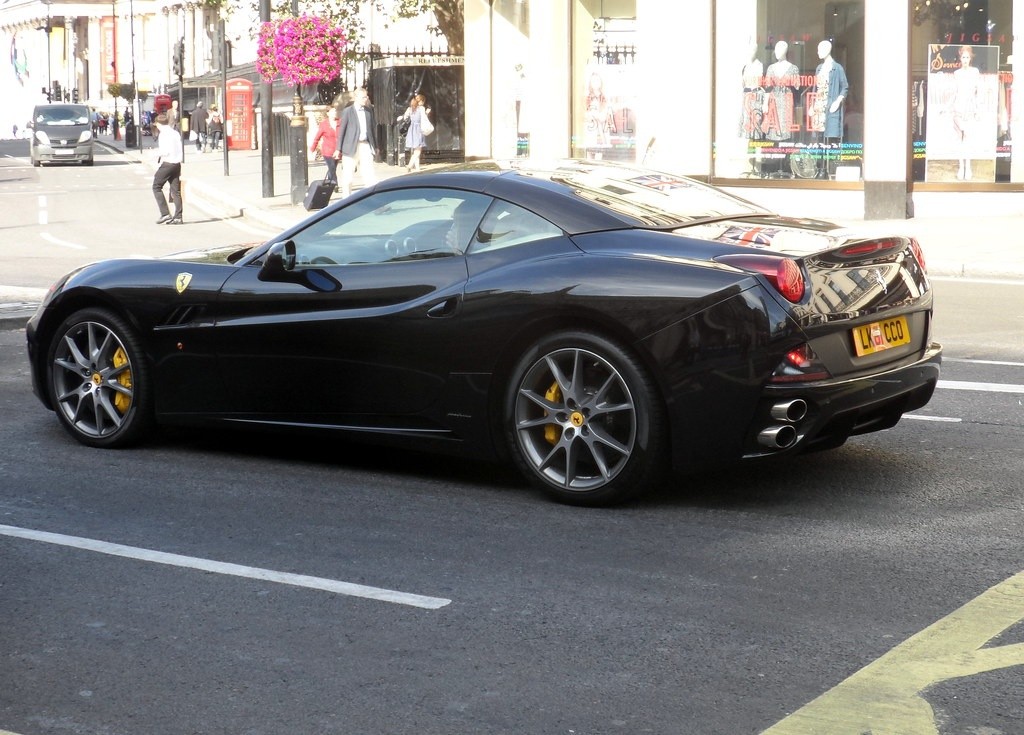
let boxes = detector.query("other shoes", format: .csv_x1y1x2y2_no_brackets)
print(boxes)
166,217,183,224
156,213,172,224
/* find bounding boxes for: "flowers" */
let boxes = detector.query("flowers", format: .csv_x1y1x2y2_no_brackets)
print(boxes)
257,16,348,87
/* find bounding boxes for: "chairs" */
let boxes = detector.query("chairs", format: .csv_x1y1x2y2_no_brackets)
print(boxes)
491,212,548,244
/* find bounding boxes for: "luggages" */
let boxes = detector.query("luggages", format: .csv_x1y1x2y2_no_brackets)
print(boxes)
303,156,339,212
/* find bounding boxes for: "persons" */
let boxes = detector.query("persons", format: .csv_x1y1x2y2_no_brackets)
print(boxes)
333,88,379,198
309,104,343,194
165,100,179,133
404,94,434,173
189,101,209,153
946,44,985,182
90,107,113,139
151,114,184,225
205,106,223,150
741,39,849,181
141,111,150,127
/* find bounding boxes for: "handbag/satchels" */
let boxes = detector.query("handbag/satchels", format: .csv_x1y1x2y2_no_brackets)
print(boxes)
189,130,199,142
398,116,411,137
421,106,434,136
169,179,182,203
